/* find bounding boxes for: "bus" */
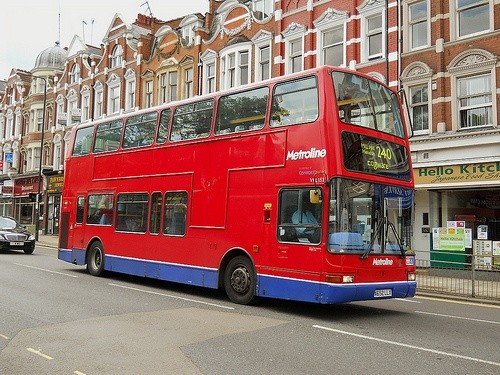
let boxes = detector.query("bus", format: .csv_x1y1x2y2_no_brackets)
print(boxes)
56,64,418,305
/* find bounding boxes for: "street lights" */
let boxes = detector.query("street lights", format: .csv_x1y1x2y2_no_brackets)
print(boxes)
15,72,47,241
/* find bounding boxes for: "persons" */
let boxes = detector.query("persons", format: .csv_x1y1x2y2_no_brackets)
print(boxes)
291,199,319,238
98,199,171,233
337,82,351,123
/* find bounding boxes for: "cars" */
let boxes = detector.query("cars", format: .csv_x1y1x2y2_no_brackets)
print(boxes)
0,216,36,255
